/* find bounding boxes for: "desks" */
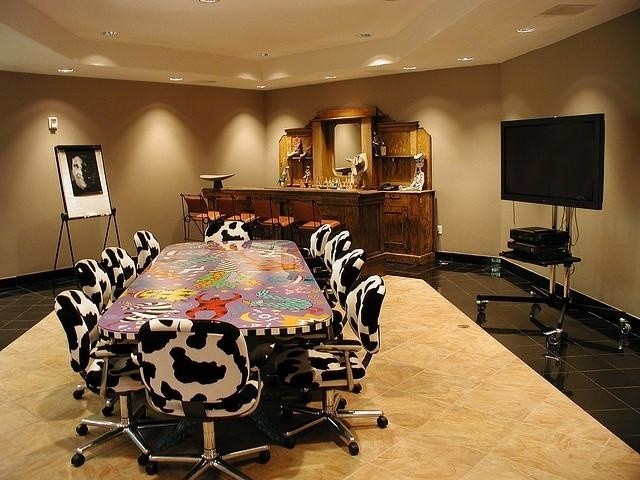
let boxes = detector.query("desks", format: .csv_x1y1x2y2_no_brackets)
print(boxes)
96,239,333,399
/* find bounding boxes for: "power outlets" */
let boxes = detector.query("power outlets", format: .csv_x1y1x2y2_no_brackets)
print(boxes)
436,225,442,235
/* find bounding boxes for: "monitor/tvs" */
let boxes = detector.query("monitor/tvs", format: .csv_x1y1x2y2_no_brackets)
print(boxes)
500,113,606,210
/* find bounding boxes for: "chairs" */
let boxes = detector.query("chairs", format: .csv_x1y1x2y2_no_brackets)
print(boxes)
275,274,387,454
205,193,263,240
310,229,352,306
134,231,160,275
132,318,271,480
73,257,147,416
180,192,226,241
204,221,252,242
252,196,303,244
289,198,344,250
101,246,139,300
54,289,181,467
299,223,333,266
272,248,366,392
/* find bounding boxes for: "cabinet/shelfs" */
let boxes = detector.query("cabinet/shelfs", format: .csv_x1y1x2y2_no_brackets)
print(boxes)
385,194,436,266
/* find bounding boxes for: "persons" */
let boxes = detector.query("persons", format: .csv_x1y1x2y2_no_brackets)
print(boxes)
372,133,380,157
276,166,288,187
70,153,101,196
303,166,312,184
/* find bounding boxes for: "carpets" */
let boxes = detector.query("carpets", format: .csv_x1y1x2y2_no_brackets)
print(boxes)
1,269,640,480
